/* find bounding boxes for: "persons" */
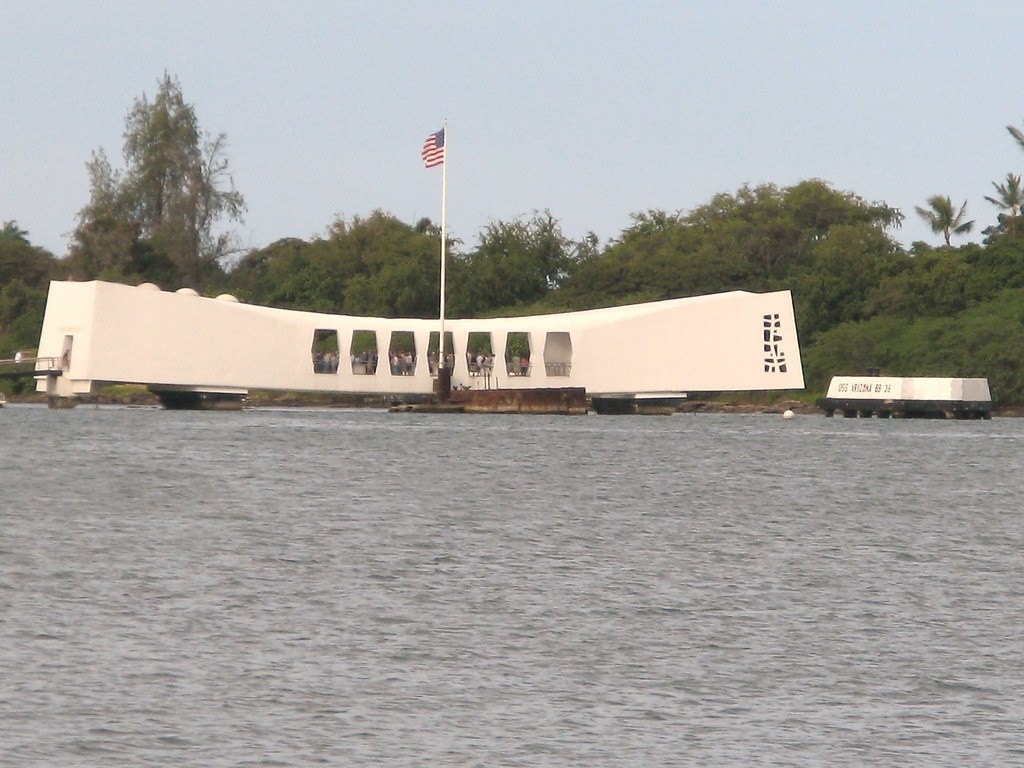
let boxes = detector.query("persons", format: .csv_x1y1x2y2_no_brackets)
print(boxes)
14,351,23,372
513,354,528,375
60,350,69,369
428,352,454,376
467,350,495,375
312,350,339,374
389,351,417,375
351,349,377,375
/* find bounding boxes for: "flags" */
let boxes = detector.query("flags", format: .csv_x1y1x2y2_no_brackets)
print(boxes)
420,128,444,167
